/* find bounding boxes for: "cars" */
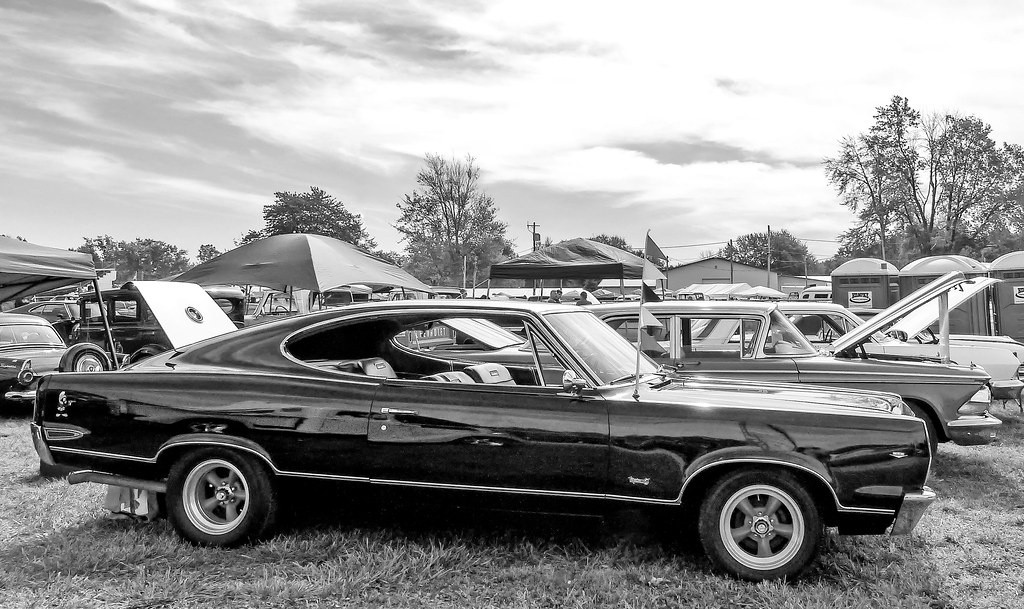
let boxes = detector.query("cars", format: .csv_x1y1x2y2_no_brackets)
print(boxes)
401,271,1024,454
0,234,904,401
27,300,940,583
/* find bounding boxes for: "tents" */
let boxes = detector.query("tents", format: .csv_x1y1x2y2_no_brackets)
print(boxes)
0,235,121,370
159,232,437,320
486,238,668,335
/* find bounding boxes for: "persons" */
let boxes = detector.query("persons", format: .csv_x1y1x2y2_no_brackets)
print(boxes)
548,290,591,306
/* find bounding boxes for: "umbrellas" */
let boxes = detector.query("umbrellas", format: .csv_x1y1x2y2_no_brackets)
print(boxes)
732,285,788,301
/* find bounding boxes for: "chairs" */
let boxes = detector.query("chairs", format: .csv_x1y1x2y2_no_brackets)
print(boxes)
420,371,475,383
357,357,398,378
463,363,516,385
